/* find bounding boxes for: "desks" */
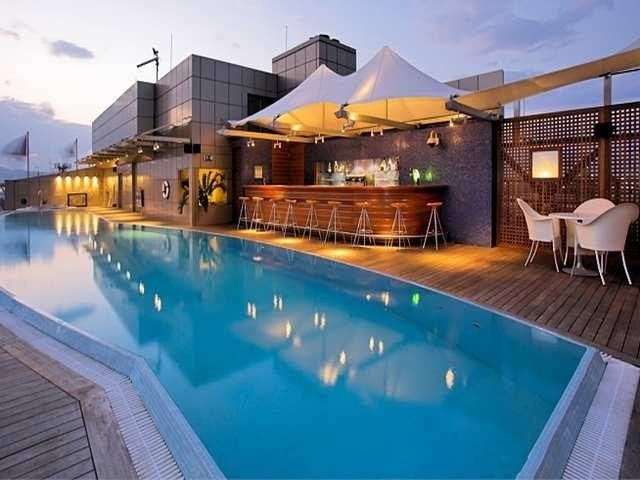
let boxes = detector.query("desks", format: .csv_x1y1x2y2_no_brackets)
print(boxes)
548,212,608,278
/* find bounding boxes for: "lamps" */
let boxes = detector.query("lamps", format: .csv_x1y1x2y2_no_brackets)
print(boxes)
243,112,390,151
86,140,161,171
527,147,564,181
424,129,441,148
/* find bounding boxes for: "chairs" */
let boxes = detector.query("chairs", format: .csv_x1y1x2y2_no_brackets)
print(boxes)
516,196,568,274
568,194,617,272
568,200,639,288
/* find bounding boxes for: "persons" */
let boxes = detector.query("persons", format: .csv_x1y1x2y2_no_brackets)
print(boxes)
0,182,6,211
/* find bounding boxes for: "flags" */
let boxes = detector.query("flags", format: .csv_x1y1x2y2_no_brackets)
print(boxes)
0,135,27,155
63,139,76,157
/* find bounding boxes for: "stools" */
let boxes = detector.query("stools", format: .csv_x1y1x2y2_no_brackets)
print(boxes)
229,195,447,251
